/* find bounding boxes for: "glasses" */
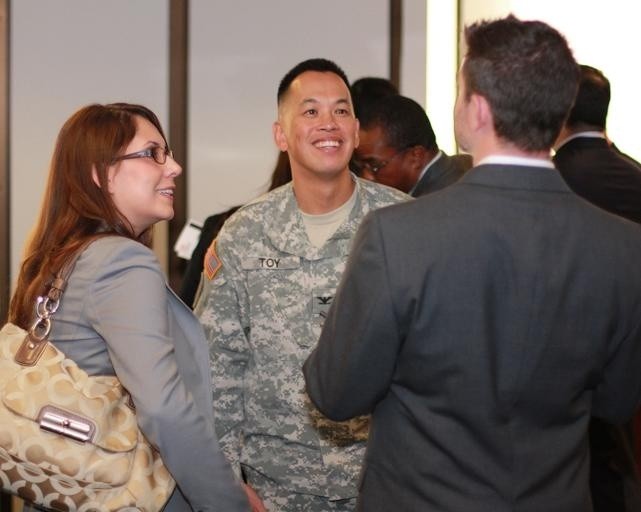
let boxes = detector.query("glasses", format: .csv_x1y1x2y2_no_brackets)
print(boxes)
352,147,411,174
113,147,174,165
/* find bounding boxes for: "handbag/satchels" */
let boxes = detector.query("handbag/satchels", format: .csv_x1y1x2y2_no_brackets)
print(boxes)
0,232,178,512
